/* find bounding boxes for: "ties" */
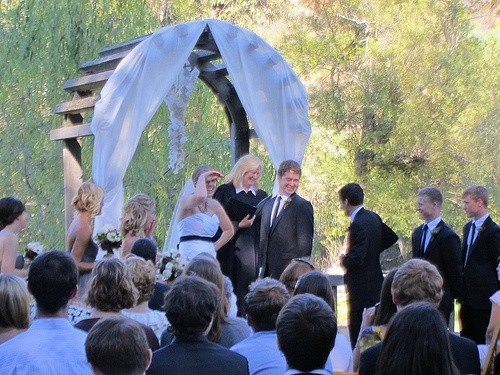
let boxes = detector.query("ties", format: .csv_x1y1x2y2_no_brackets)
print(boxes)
466,222,476,266
420,224,428,258
272,195,282,226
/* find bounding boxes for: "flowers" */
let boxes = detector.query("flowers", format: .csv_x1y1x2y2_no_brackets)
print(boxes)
158,252,187,283
93,225,123,258
358,325,386,353
284,197,292,210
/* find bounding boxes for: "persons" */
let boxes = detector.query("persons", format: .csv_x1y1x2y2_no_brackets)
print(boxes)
121,194,156,256
0,239,353,375
0,197,27,276
457,186,500,345
375,301,459,375
358,258,481,375
338,182,399,349
66,181,114,299
353,269,398,372
213,154,268,318
162,166,234,261
486,257,500,345
259,160,314,280
412,188,460,327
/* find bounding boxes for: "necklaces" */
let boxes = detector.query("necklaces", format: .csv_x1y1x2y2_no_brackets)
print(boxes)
198,202,207,214
